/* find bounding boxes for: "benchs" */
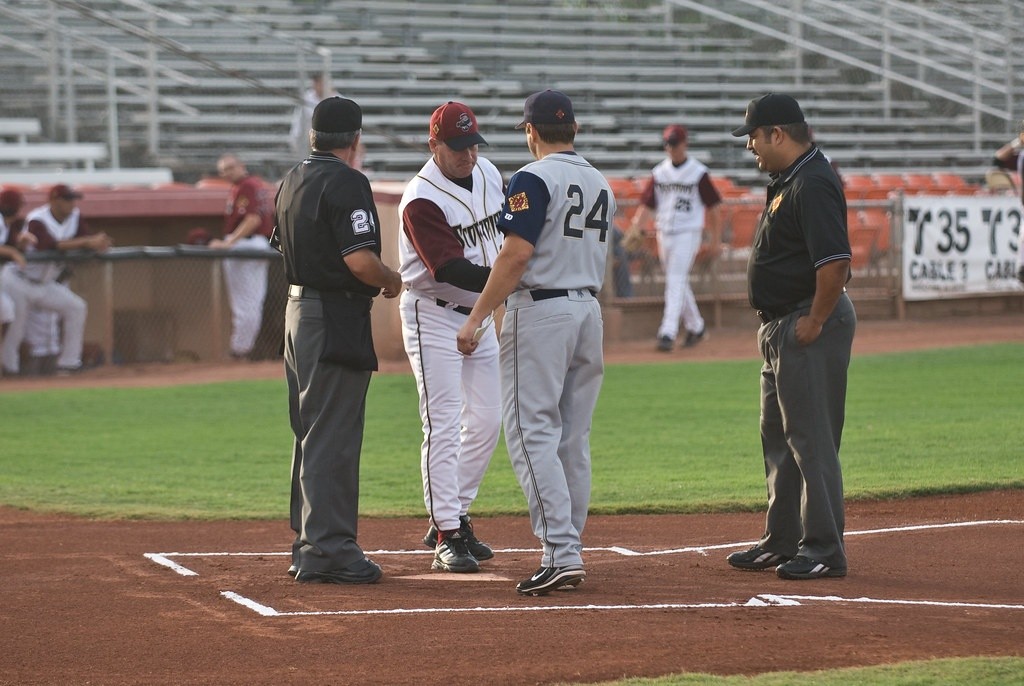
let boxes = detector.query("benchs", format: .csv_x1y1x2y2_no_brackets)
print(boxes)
0,0,1024,189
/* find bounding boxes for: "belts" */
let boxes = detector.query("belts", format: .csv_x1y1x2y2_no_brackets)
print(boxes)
437,300,473,315
288,285,323,299
756,297,813,325
530,289,597,301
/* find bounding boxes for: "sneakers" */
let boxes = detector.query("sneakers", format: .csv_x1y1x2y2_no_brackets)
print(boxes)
431,531,481,573
516,566,586,596
775,555,847,580
423,515,494,561
727,544,791,572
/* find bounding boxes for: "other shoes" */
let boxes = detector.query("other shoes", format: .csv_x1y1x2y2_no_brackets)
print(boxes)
657,336,673,352
53,368,91,377
681,328,705,348
288,565,299,577
294,558,384,584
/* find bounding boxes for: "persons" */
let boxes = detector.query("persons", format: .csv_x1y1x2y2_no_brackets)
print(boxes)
0,184,113,375
275,97,402,582
622,124,724,354
394,100,507,573
456,88,618,595
990,133,1024,282
208,155,273,360
724,92,857,579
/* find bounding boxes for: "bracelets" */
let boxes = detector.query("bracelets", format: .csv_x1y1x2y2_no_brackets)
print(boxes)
1011,138,1022,151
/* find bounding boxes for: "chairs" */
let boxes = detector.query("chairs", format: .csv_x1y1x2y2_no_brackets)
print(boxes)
606,173,1024,295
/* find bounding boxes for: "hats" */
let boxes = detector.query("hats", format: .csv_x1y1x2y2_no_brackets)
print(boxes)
732,92,804,137
50,185,83,201
515,89,574,130
430,102,489,151
312,96,362,132
0,186,24,214
664,125,687,144
